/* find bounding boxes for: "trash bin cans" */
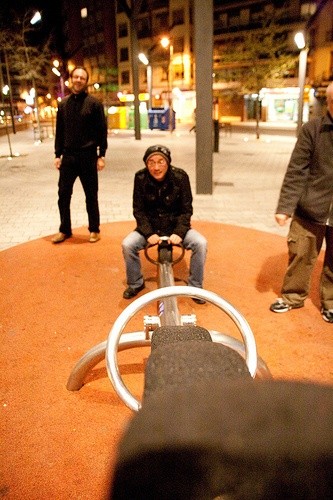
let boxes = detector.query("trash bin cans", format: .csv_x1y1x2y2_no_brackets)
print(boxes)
147,105,176,131
128,101,149,129
107,105,128,128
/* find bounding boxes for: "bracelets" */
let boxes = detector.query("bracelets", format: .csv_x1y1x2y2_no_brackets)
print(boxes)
98,157,105,160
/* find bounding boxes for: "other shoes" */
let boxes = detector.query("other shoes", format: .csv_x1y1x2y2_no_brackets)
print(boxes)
123,281,145,299
192,298,206,304
51,232,67,242
88,232,100,242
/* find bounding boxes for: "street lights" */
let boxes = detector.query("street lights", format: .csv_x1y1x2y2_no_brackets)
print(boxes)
159,36,175,106
137,51,155,109
293,30,311,135
26,9,44,141
51,60,67,99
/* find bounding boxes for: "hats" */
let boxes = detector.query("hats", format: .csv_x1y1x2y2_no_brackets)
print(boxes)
142,144,171,165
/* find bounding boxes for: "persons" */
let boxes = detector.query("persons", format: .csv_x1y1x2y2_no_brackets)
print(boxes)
270,81,333,323
121,144,208,304
52,66,108,243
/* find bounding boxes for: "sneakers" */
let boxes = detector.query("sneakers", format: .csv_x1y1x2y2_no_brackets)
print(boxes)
321,305,333,323
270,297,305,312
